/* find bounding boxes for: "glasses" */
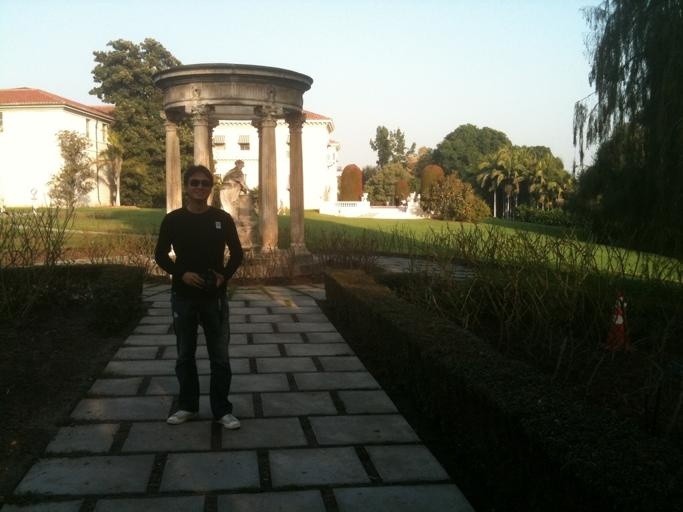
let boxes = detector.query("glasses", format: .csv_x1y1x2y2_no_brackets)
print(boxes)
188,178,213,188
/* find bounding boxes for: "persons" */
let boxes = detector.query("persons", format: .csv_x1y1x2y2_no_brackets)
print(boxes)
154,164,243,429
219,159,249,227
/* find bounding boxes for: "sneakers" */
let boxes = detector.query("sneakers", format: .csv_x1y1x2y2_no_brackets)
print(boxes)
166,410,199,425
218,414,240,430
